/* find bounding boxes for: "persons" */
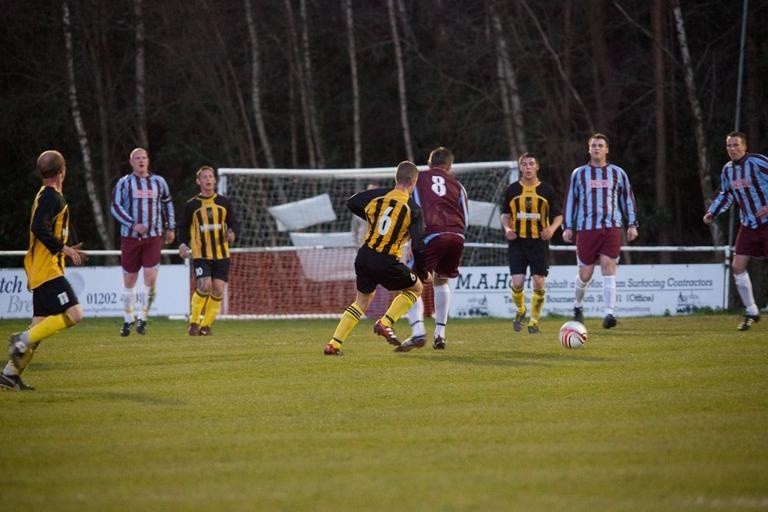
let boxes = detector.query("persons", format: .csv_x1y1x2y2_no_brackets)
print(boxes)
393,148,471,352
703,130,768,331
498,152,564,333
0,151,89,392
109,147,177,336
180,166,240,336
322,162,433,354
562,133,639,330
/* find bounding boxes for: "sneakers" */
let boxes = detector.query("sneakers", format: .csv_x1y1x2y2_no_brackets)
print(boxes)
200,325,213,335
323,343,343,355
528,324,541,333
395,335,426,352
374,320,400,345
603,313,616,328
136,317,147,335
9,331,26,369
0,373,35,392
737,312,761,331
120,320,136,337
433,336,447,348
513,305,527,331
188,322,200,336
573,305,585,323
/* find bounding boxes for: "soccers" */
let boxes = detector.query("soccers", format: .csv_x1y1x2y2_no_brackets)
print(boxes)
559,320,587,349
